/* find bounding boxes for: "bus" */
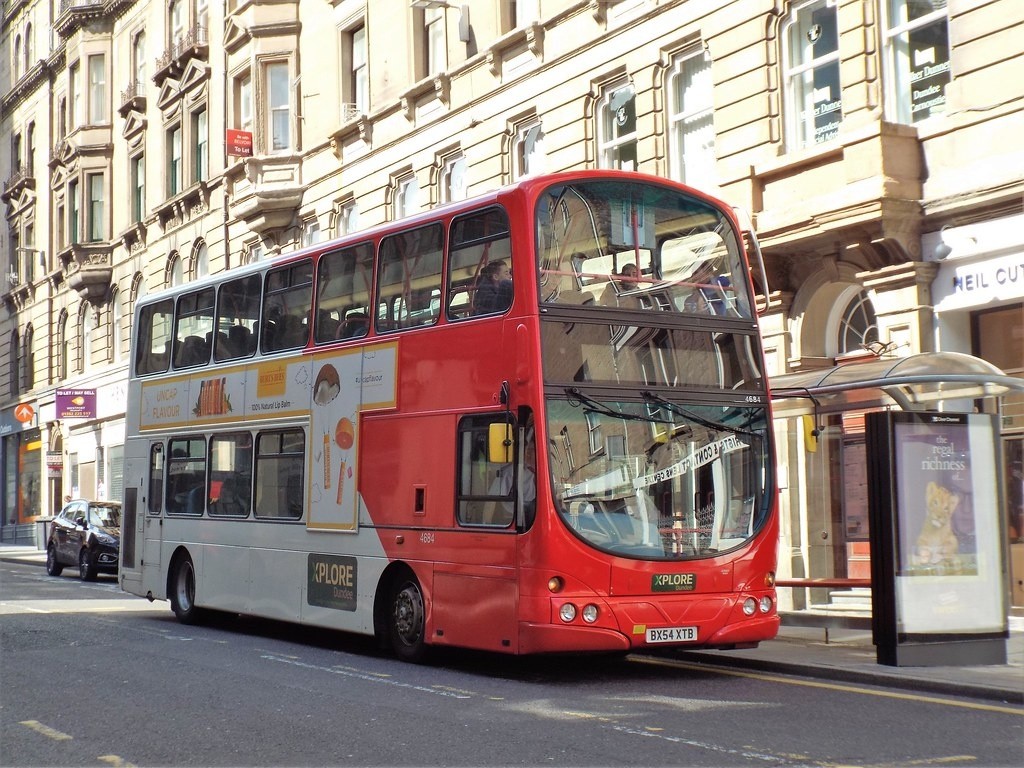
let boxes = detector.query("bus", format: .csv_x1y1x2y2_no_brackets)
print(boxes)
115,166,828,665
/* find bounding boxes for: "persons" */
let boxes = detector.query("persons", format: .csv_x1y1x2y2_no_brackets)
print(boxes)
153,254,732,526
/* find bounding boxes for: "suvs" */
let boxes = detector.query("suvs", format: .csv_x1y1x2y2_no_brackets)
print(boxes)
44,496,123,583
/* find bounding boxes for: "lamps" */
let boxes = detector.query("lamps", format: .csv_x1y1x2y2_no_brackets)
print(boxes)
935,224,976,260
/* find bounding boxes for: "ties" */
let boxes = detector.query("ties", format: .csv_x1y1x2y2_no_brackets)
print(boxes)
527,466,536,475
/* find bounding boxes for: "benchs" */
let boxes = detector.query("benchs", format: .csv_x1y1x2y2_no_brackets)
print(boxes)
185,470,238,488
335,319,406,340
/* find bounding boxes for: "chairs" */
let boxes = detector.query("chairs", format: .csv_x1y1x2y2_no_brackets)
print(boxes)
253,321,275,353
222,326,251,358
186,484,210,514
272,315,303,351
301,309,331,345
346,312,369,338
175,336,205,366
200,331,227,362
160,339,183,370
172,473,193,512
